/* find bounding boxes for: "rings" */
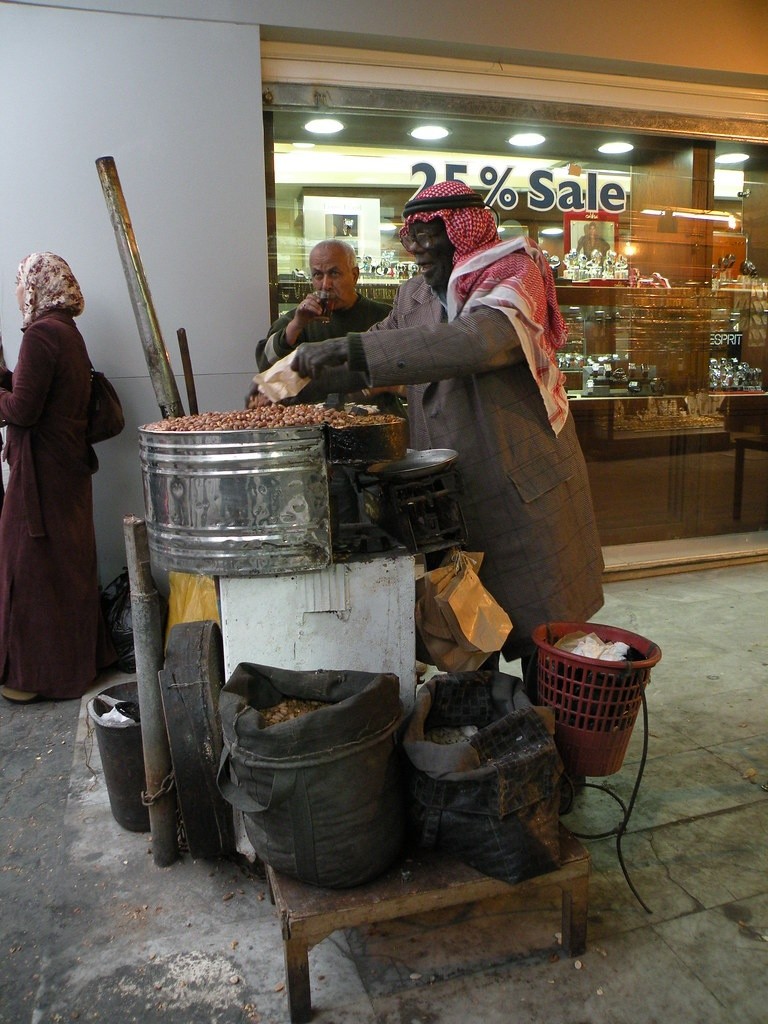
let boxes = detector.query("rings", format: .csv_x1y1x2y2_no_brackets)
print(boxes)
303,303,306,310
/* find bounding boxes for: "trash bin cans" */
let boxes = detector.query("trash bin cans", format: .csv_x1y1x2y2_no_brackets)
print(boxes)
86,679,151,832
531,620,662,778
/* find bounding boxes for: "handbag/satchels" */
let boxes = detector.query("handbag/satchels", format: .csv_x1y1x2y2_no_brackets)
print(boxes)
88,361,125,444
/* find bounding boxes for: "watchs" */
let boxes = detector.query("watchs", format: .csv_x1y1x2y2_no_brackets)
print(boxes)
278,249,768,431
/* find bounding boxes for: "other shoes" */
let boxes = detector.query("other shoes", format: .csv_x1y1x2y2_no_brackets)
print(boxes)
2,686,39,702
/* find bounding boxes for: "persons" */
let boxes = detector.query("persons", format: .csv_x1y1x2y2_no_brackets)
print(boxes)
0,250,99,705
244,180,608,704
255,239,409,419
577,222,610,261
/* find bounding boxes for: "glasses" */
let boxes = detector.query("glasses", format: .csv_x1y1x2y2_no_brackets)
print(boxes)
400,230,445,252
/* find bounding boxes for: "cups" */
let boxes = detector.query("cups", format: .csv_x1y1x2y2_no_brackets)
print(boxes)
310,290,339,324
